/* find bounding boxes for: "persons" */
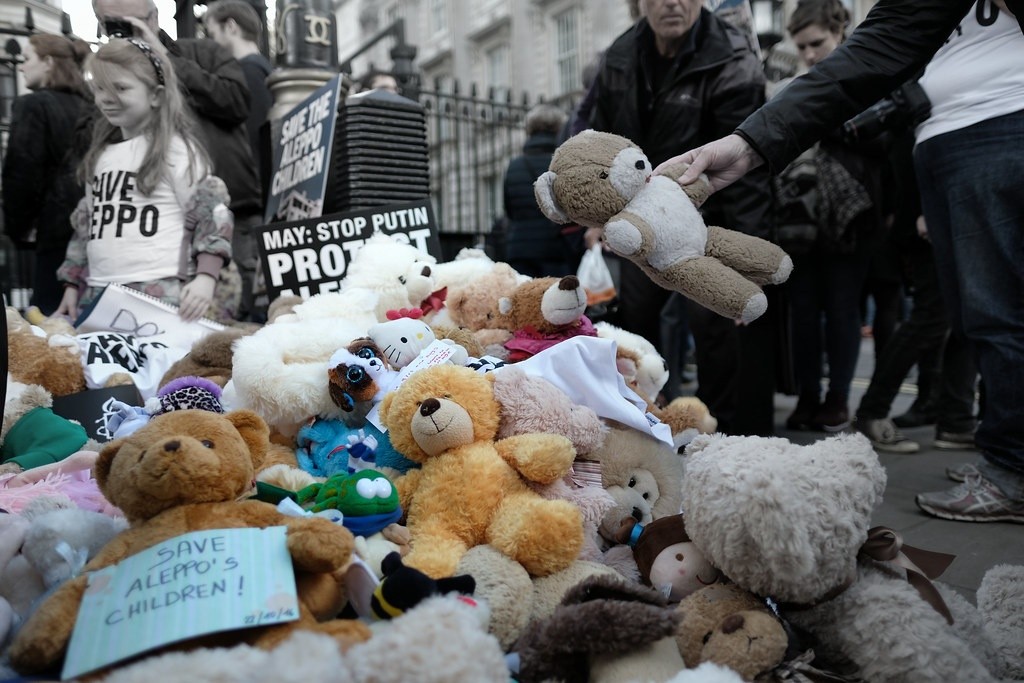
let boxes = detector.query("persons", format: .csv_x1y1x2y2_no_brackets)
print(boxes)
0,0,276,322
651,1,1024,522
503,0,979,454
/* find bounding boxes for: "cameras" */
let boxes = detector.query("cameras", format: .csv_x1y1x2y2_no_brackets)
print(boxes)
844,80,932,143
105,19,134,40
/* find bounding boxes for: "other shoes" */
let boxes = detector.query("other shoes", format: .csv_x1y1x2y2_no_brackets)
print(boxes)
892,410,934,428
786,404,849,431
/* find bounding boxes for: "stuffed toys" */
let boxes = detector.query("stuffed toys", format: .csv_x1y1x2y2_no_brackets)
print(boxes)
379,363,583,579
0,231,746,682
5,408,369,681
532,128,792,323
672,582,859,683
681,431,1024,683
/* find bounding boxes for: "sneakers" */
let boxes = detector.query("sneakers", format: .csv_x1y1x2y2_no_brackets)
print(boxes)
852,413,919,454
915,464,1024,522
932,423,982,450
